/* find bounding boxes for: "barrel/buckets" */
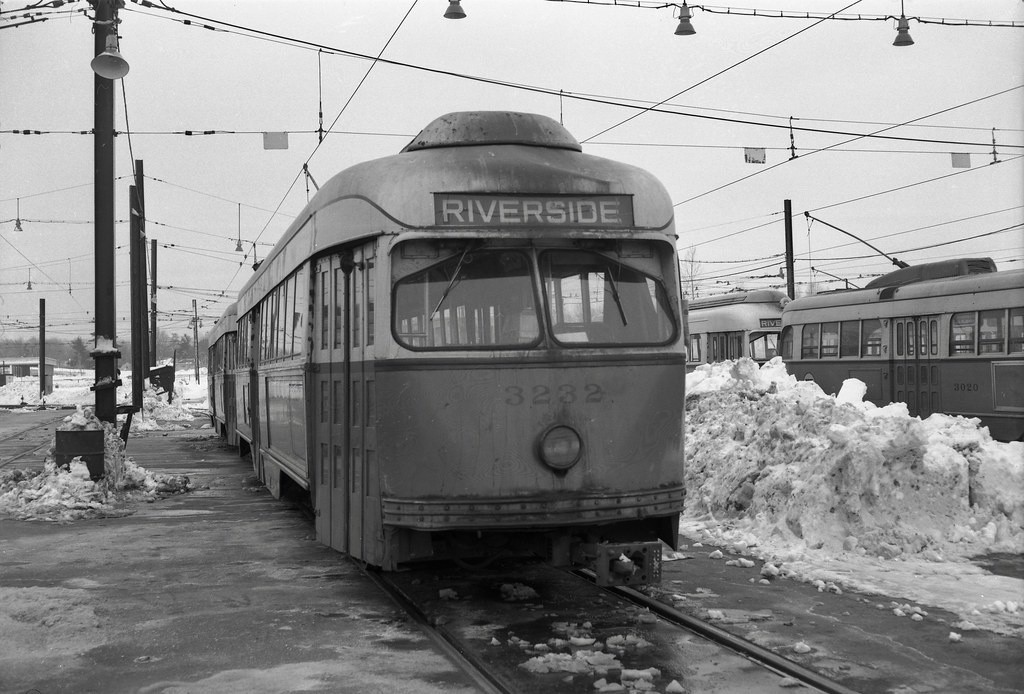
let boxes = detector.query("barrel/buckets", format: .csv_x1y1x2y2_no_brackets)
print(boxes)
56,430,104,482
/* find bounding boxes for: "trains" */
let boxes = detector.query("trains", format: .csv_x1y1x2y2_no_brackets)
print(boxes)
592,257,1024,442
206,110,690,590
